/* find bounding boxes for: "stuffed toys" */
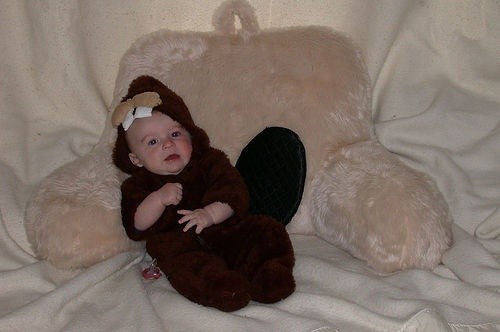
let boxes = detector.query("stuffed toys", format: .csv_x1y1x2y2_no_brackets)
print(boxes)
24,0,455,276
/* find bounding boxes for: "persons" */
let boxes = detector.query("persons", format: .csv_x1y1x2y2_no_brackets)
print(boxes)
111,75,297,312
